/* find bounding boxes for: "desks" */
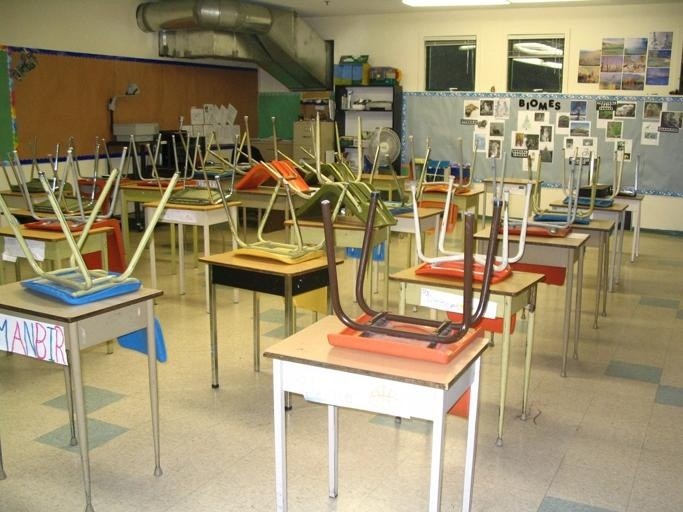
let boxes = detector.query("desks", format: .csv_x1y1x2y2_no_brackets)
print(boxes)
264,317,490,511
474,226,590,376
388,269,545,446
0,276,164,512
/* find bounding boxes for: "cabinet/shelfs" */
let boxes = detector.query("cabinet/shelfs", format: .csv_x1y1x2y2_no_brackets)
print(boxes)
334,85,402,176
